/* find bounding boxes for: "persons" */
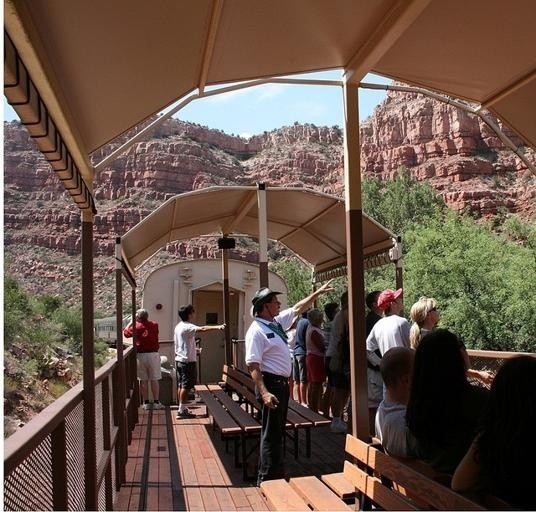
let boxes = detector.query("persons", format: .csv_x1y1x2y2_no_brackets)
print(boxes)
288,303,340,421
373,296,535,511
124,309,165,410
329,290,407,445
244,278,335,487
173,303,226,419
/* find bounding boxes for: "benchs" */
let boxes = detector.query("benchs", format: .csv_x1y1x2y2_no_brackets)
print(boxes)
259,434,487,511
194,365,262,482
283,397,332,460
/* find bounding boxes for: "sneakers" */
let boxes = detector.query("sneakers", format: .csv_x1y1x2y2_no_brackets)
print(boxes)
142,402,152,411
176,408,197,419
153,401,165,410
331,420,347,434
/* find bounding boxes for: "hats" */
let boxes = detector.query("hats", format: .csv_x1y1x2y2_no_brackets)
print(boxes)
377,288,403,310
251,287,283,316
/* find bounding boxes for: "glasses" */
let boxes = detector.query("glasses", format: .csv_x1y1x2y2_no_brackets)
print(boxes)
428,306,437,314
